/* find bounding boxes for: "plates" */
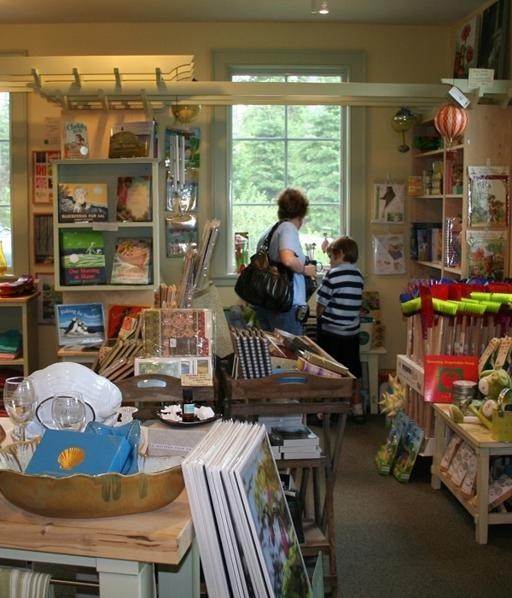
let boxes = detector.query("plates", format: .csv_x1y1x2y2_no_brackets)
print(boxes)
158,411,222,428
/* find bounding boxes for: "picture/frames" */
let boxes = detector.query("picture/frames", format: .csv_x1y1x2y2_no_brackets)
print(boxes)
55,303,107,346
105,303,152,342
34,272,62,326
32,210,54,265
451,11,480,79
32,149,62,205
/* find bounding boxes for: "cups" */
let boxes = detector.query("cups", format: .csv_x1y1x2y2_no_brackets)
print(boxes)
452,381,478,408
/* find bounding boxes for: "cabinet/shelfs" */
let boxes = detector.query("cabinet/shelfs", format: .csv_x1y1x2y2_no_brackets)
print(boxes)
217,334,357,598
0,287,41,415
51,158,160,292
116,384,222,426
405,99,512,281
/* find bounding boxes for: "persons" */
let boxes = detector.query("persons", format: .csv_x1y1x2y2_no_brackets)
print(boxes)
257,188,316,337
315,235,366,423
380,187,396,217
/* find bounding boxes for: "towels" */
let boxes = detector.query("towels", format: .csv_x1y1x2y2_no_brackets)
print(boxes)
0,565,54,598
0,329,22,360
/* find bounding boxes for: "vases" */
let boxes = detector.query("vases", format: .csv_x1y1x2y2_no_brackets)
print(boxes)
357,322,373,352
0,240,6,275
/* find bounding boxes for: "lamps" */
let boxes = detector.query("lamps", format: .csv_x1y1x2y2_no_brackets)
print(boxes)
318,0,331,15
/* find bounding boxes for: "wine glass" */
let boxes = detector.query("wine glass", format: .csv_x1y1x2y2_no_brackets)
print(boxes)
3,376,36,441
52,392,86,432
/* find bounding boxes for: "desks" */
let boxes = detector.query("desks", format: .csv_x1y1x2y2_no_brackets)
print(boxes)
0,484,204,598
360,345,388,415
56,337,118,369
430,403,512,544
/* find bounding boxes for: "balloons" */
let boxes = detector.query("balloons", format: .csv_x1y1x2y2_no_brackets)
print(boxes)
434,104,468,147
171,103,202,123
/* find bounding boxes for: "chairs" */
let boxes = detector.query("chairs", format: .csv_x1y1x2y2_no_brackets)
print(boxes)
113,373,183,427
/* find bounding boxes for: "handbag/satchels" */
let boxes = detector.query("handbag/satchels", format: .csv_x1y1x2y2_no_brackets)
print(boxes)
233,250,294,312
